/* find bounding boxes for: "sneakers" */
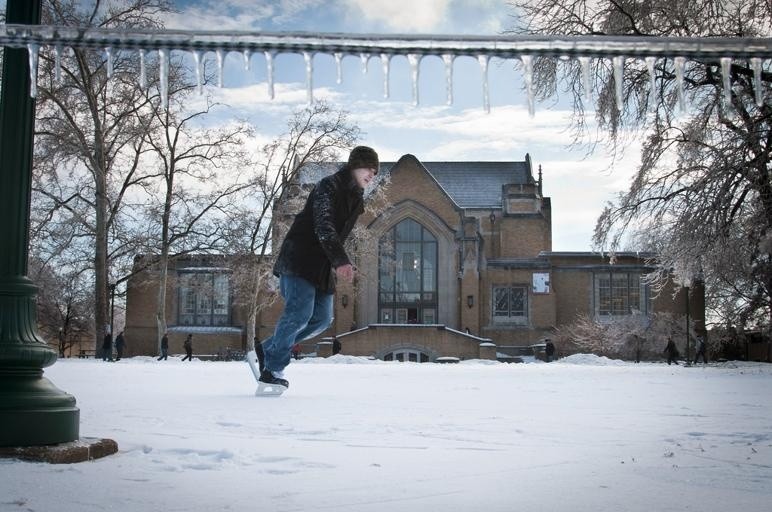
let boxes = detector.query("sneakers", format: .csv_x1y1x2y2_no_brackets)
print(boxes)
258,368,290,389
253,336,270,379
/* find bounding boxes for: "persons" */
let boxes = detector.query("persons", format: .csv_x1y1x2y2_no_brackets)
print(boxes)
332,336,341,355
115,332,127,361
692,336,708,364
181,334,193,361
157,334,168,361
466,328,470,334
103,332,113,362
545,340,554,362
244,146,380,395
663,338,679,365
293,344,301,359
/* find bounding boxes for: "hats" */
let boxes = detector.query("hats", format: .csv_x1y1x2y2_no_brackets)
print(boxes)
347,145,380,173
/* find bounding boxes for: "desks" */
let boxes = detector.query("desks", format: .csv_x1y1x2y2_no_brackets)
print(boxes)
77,349,98,353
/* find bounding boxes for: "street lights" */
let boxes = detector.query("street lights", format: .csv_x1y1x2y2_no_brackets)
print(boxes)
107,273,118,362
59,326,62,358
683,278,693,366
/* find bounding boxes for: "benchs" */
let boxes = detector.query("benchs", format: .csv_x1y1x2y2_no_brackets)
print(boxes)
73,353,97,357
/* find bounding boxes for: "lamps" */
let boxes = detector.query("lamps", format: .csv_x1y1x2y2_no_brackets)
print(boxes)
466,293,475,309
340,292,349,309
